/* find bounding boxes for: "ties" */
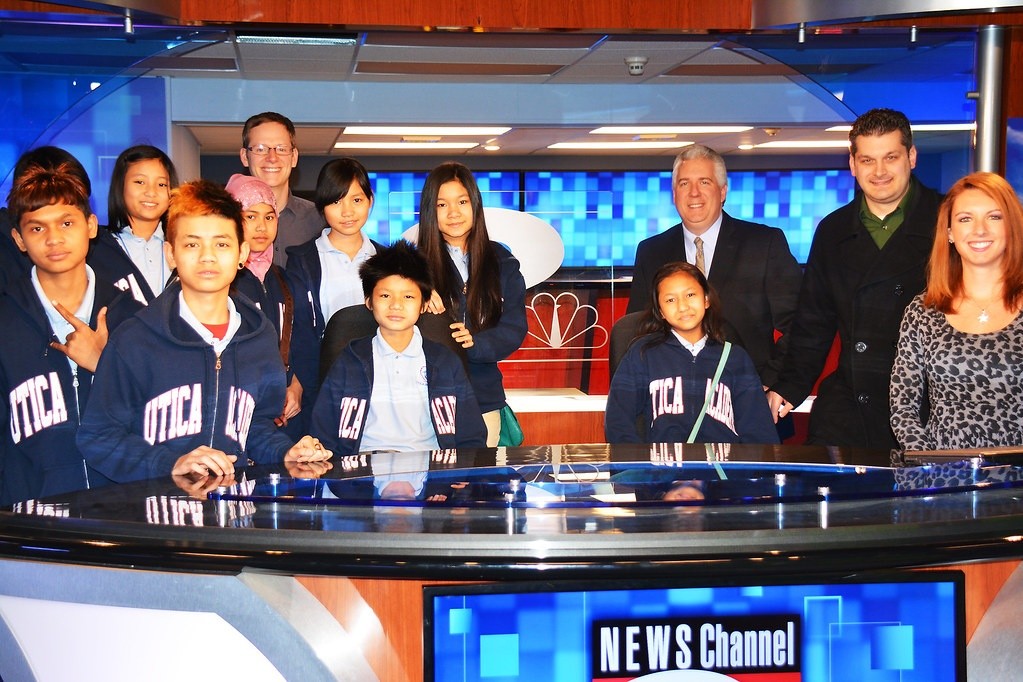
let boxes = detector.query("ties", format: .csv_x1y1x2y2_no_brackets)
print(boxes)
694,237,706,277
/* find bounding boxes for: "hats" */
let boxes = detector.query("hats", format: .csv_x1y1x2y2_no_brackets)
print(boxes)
224,174,278,219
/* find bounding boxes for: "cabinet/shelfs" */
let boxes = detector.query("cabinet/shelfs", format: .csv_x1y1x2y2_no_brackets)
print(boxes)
508,396,816,447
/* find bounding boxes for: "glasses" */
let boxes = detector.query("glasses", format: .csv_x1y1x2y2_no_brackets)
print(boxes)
247,143,293,156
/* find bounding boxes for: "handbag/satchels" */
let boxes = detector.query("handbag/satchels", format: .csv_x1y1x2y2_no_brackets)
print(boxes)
497,405,524,447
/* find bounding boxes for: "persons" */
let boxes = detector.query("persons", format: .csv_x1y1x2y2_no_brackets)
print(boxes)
626,143,802,446
0,112,529,508
605,262,778,444
765,109,944,450
891,173,1023,450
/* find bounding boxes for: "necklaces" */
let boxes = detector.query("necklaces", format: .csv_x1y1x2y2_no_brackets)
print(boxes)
962,281,1005,324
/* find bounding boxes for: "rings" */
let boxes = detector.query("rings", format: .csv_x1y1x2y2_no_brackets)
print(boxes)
314,443,321,450
